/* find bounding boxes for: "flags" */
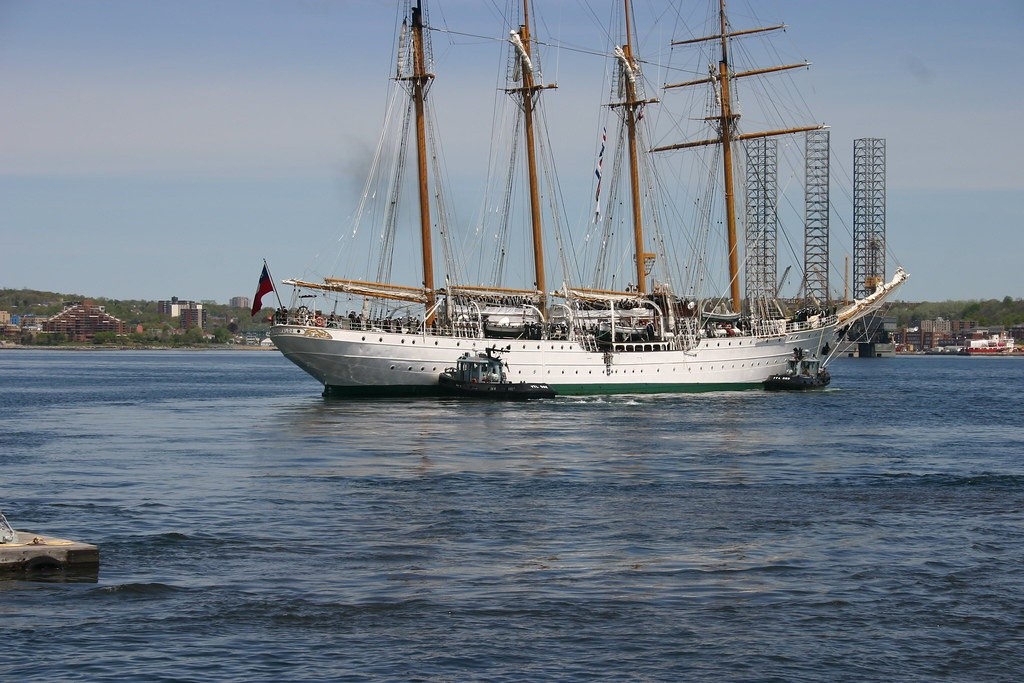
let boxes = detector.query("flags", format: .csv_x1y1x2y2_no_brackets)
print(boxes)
251,264,273,317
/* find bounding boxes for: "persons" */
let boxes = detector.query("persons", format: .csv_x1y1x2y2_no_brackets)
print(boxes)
737,317,751,331
329,310,419,333
796,304,837,321
276,306,288,324
646,321,655,340
482,317,490,338
524,321,596,340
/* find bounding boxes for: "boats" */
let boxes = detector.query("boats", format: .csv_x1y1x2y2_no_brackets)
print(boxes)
762,346,830,391
436,347,560,399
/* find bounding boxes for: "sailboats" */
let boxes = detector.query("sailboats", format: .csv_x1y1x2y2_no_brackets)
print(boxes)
255,1,922,394
